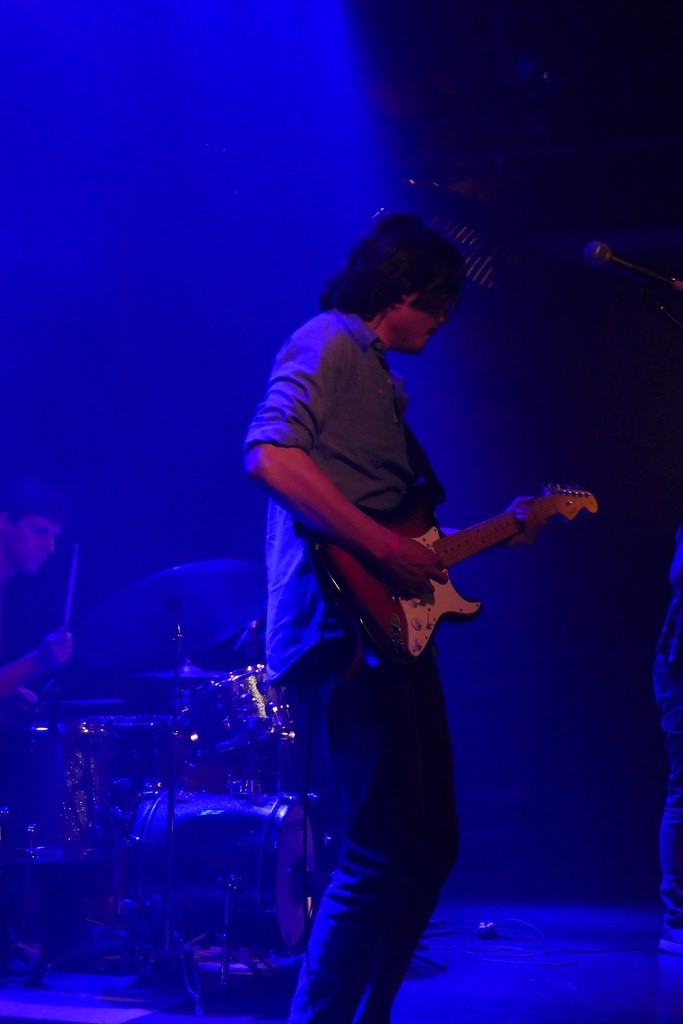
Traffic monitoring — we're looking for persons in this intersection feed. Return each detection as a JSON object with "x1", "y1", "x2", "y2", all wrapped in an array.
[
  {"x1": 651, "y1": 519, "x2": 683, "y2": 953},
  {"x1": 0, "y1": 500, "x2": 73, "y2": 838},
  {"x1": 243, "y1": 214, "x2": 471, "y2": 1023}
]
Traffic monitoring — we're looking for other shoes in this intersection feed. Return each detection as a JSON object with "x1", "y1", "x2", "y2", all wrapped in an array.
[{"x1": 659, "y1": 922, "x2": 683, "y2": 955}]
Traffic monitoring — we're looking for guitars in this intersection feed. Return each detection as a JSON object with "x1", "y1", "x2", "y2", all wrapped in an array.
[{"x1": 301, "y1": 482, "x2": 605, "y2": 682}]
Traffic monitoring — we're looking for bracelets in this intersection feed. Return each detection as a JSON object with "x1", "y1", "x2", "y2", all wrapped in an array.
[{"x1": 385, "y1": 549, "x2": 393, "y2": 564}]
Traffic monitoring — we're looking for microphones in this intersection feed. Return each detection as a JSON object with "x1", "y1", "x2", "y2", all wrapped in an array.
[{"x1": 583, "y1": 241, "x2": 683, "y2": 291}]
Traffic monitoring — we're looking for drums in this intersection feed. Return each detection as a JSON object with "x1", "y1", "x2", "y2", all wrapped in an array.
[
  {"x1": 0, "y1": 845, "x2": 138, "y2": 952},
  {"x1": 117, "y1": 780, "x2": 323, "y2": 956},
  {"x1": 170, "y1": 662, "x2": 306, "y2": 768}
]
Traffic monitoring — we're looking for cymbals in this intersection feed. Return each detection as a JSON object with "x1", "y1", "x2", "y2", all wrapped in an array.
[{"x1": 126, "y1": 665, "x2": 236, "y2": 687}]
